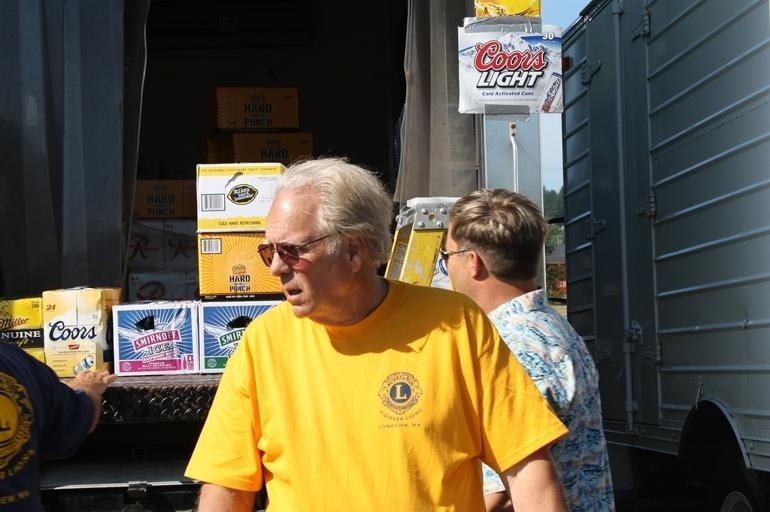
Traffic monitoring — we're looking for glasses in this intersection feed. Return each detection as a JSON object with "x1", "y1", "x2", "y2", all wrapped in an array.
[
  {"x1": 439, "y1": 247, "x2": 469, "y2": 260},
  {"x1": 257, "y1": 235, "x2": 330, "y2": 267}
]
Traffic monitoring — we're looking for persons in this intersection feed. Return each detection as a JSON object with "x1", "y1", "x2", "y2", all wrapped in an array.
[
  {"x1": 0, "y1": 341, "x2": 118, "y2": 512},
  {"x1": 443, "y1": 187, "x2": 616, "y2": 512},
  {"x1": 184, "y1": 154, "x2": 572, "y2": 512}
]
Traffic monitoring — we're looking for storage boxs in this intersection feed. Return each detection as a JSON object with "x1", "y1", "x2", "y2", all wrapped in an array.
[
  {"x1": 2, "y1": 296, "x2": 43, "y2": 377},
  {"x1": 187, "y1": 273, "x2": 206, "y2": 301},
  {"x1": 43, "y1": 284, "x2": 123, "y2": 378},
  {"x1": 110, "y1": 299, "x2": 199, "y2": 376},
  {"x1": 198, "y1": 301, "x2": 283, "y2": 375},
  {"x1": 195, "y1": 160, "x2": 289, "y2": 234},
  {"x1": 208, "y1": 86, "x2": 300, "y2": 134},
  {"x1": 202, "y1": 133, "x2": 312, "y2": 168},
  {"x1": 133, "y1": 179, "x2": 195, "y2": 219},
  {"x1": 161, "y1": 219, "x2": 195, "y2": 270},
  {"x1": 128, "y1": 271, "x2": 185, "y2": 303},
  {"x1": 196, "y1": 230, "x2": 285, "y2": 295},
  {"x1": 125, "y1": 220, "x2": 163, "y2": 270}
]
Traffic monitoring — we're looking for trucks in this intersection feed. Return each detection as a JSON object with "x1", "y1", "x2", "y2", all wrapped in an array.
[{"x1": 556, "y1": 2, "x2": 770, "y2": 511}]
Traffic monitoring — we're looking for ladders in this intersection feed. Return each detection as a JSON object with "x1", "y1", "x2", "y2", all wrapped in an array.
[{"x1": 382, "y1": 201, "x2": 459, "y2": 294}]
